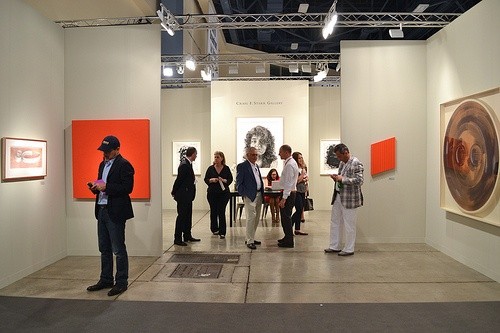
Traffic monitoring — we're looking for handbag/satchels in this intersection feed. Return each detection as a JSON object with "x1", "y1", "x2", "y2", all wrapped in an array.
[{"x1": 303, "y1": 196, "x2": 314, "y2": 211}]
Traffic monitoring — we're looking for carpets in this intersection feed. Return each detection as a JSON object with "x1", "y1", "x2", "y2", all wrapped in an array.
[{"x1": 0, "y1": 294, "x2": 500, "y2": 333}]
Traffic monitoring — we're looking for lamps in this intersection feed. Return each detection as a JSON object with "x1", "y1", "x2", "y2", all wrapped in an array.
[
  {"x1": 389, "y1": 23, "x2": 404, "y2": 38},
  {"x1": 229, "y1": 64, "x2": 240, "y2": 75},
  {"x1": 163, "y1": 64, "x2": 174, "y2": 77},
  {"x1": 200, "y1": 65, "x2": 214, "y2": 82},
  {"x1": 412, "y1": 4, "x2": 429, "y2": 13},
  {"x1": 185, "y1": 56, "x2": 197, "y2": 71},
  {"x1": 302, "y1": 62, "x2": 312, "y2": 72},
  {"x1": 297, "y1": 4, "x2": 309, "y2": 14},
  {"x1": 291, "y1": 43, "x2": 298, "y2": 51},
  {"x1": 156, "y1": 6, "x2": 178, "y2": 36},
  {"x1": 288, "y1": 62, "x2": 298, "y2": 73},
  {"x1": 321, "y1": 0, "x2": 338, "y2": 39},
  {"x1": 256, "y1": 63, "x2": 266, "y2": 74}
]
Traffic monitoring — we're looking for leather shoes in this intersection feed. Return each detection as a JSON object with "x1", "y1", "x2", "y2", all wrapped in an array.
[
  {"x1": 87, "y1": 279, "x2": 114, "y2": 291},
  {"x1": 108, "y1": 282, "x2": 125, "y2": 296}
]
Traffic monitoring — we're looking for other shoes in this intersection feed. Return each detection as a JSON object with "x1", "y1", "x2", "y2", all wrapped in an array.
[
  {"x1": 213, "y1": 230, "x2": 219, "y2": 235},
  {"x1": 277, "y1": 239, "x2": 294, "y2": 248},
  {"x1": 174, "y1": 241, "x2": 188, "y2": 246},
  {"x1": 245, "y1": 240, "x2": 261, "y2": 245},
  {"x1": 247, "y1": 243, "x2": 257, "y2": 249},
  {"x1": 220, "y1": 234, "x2": 225, "y2": 239},
  {"x1": 184, "y1": 237, "x2": 200, "y2": 242},
  {"x1": 338, "y1": 251, "x2": 354, "y2": 256},
  {"x1": 294, "y1": 230, "x2": 308, "y2": 235},
  {"x1": 301, "y1": 219, "x2": 306, "y2": 222},
  {"x1": 324, "y1": 248, "x2": 341, "y2": 253}
]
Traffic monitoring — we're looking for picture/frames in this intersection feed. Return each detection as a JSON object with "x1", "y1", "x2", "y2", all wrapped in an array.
[
  {"x1": 318, "y1": 139, "x2": 342, "y2": 176},
  {"x1": 172, "y1": 140, "x2": 202, "y2": 176},
  {"x1": 1, "y1": 137, "x2": 48, "y2": 181}
]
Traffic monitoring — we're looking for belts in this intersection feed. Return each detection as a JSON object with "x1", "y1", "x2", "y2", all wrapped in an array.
[{"x1": 336, "y1": 191, "x2": 341, "y2": 194}]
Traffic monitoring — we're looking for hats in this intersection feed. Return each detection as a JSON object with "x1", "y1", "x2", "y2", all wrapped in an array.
[{"x1": 97, "y1": 135, "x2": 121, "y2": 153}]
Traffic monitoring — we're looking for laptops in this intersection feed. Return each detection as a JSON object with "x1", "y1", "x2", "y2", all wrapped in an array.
[{"x1": 271, "y1": 181, "x2": 280, "y2": 190}]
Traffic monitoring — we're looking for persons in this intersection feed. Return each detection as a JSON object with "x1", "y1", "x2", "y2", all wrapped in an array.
[
  {"x1": 236, "y1": 146, "x2": 265, "y2": 250},
  {"x1": 171, "y1": 147, "x2": 201, "y2": 246},
  {"x1": 87, "y1": 136, "x2": 136, "y2": 296},
  {"x1": 278, "y1": 145, "x2": 298, "y2": 248},
  {"x1": 292, "y1": 152, "x2": 309, "y2": 235},
  {"x1": 204, "y1": 151, "x2": 233, "y2": 239},
  {"x1": 324, "y1": 143, "x2": 364, "y2": 256},
  {"x1": 262, "y1": 168, "x2": 282, "y2": 222}
]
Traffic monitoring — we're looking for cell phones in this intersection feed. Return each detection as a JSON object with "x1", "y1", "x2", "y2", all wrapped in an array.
[{"x1": 87, "y1": 183, "x2": 95, "y2": 188}]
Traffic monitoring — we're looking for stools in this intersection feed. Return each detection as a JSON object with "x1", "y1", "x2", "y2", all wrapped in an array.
[{"x1": 235, "y1": 203, "x2": 245, "y2": 222}]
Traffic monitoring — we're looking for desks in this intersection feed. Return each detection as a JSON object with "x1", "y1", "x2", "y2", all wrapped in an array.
[{"x1": 229, "y1": 190, "x2": 283, "y2": 227}]
